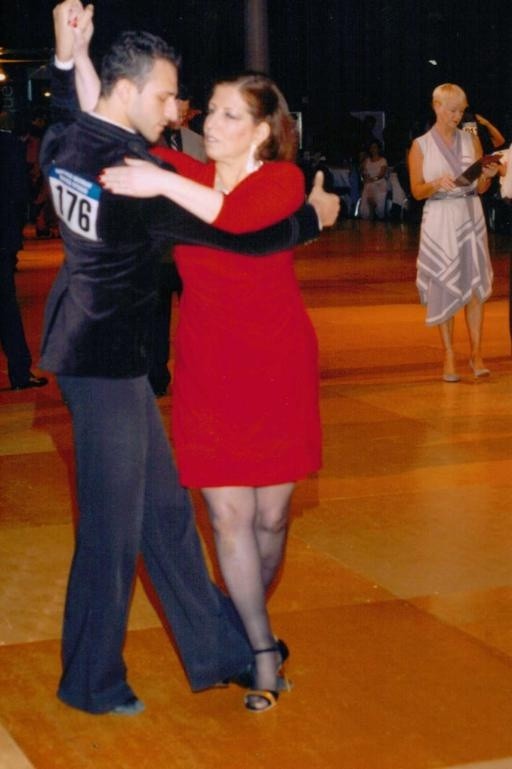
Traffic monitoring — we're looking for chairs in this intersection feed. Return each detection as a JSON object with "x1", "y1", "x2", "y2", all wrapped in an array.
[{"x1": 384, "y1": 173, "x2": 411, "y2": 235}]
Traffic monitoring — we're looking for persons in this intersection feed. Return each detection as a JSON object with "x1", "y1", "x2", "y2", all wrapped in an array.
[
  {"x1": 407, "y1": 84, "x2": 512, "y2": 382},
  {"x1": 152, "y1": 70, "x2": 210, "y2": 165},
  {"x1": 359, "y1": 142, "x2": 388, "y2": 219},
  {"x1": 1, "y1": 131, "x2": 48, "y2": 389}
]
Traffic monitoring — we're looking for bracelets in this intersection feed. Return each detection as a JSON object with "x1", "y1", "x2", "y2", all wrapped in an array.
[{"x1": 374, "y1": 176, "x2": 378, "y2": 181}]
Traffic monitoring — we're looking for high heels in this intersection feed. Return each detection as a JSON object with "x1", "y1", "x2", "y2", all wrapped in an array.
[
  {"x1": 243, "y1": 638, "x2": 289, "y2": 713},
  {"x1": 442, "y1": 359, "x2": 490, "y2": 382}
]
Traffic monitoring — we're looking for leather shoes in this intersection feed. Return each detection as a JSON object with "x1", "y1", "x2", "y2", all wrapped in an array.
[
  {"x1": 114, "y1": 695, "x2": 144, "y2": 714},
  {"x1": 10, "y1": 372, "x2": 47, "y2": 390},
  {"x1": 216, "y1": 657, "x2": 293, "y2": 692}
]
